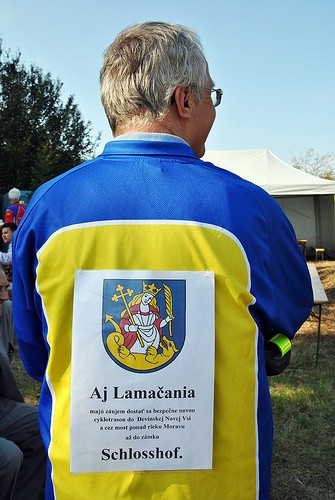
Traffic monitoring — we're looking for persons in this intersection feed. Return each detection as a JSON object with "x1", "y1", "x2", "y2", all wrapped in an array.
[
  {"x1": 0, "y1": 187, "x2": 27, "y2": 283},
  {"x1": 0, "y1": 268, "x2": 50, "y2": 500},
  {"x1": 10, "y1": 20, "x2": 314, "y2": 500}
]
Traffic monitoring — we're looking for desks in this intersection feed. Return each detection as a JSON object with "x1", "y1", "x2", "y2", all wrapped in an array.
[
  {"x1": 308, "y1": 300, "x2": 327, "y2": 366},
  {"x1": 297, "y1": 239, "x2": 309, "y2": 259}
]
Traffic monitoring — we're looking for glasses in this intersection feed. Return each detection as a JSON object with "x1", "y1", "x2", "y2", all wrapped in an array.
[{"x1": 170, "y1": 85, "x2": 223, "y2": 107}]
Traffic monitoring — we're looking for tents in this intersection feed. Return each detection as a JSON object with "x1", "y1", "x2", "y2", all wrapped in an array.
[{"x1": 197, "y1": 149, "x2": 335, "y2": 261}]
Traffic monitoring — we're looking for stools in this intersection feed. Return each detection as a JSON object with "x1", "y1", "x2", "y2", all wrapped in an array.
[{"x1": 310, "y1": 248, "x2": 325, "y2": 260}]
